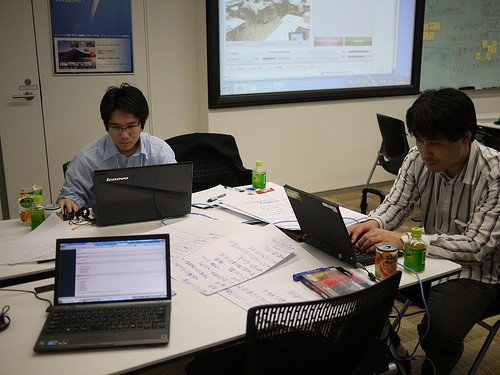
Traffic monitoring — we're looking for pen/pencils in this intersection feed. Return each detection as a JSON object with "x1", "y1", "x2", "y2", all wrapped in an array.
[{"x1": 207, "y1": 193, "x2": 226, "y2": 202}]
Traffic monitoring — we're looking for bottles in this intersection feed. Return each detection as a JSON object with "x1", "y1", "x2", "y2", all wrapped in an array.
[
  {"x1": 403, "y1": 227, "x2": 427, "y2": 274},
  {"x1": 252, "y1": 161, "x2": 266, "y2": 190}
]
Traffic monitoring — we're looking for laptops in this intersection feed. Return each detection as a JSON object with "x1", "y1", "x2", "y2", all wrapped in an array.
[
  {"x1": 91, "y1": 161, "x2": 193, "y2": 226},
  {"x1": 283, "y1": 184, "x2": 404, "y2": 267},
  {"x1": 33, "y1": 232, "x2": 172, "y2": 352}
]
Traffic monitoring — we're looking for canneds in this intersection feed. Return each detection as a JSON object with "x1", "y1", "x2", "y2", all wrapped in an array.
[{"x1": 376, "y1": 244, "x2": 398, "y2": 281}]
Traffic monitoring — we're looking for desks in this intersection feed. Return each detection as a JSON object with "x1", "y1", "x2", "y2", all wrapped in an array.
[{"x1": 0, "y1": 181, "x2": 462, "y2": 375}]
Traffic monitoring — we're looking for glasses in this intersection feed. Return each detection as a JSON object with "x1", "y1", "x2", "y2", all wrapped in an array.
[{"x1": 107, "y1": 118, "x2": 143, "y2": 134}]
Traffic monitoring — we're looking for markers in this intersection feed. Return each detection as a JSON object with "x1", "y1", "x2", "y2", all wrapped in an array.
[{"x1": 293, "y1": 265, "x2": 334, "y2": 281}]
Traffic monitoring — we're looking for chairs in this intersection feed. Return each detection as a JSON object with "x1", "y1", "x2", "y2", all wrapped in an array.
[
  {"x1": 163, "y1": 132, "x2": 253, "y2": 193},
  {"x1": 359, "y1": 113, "x2": 411, "y2": 215},
  {"x1": 391, "y1": 281, "x2": 500, "y2": 375},
  {"x1": 184, "y1": 271, "x2": 403, "y2": 375}
]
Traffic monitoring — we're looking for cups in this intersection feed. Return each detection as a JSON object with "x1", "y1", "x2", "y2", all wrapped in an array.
[{"x1": 30, "y1": 205, "x2": 45, "y2": 229}]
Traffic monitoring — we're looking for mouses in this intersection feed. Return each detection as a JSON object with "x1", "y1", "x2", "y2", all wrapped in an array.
[{"x1": 62, "y1": 205, "x2": 73, "y2": 220}]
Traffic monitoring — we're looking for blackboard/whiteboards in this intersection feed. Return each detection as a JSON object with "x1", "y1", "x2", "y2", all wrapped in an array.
[{"x1": 418, "y1": 0, "x2": 500, "y2": 96}]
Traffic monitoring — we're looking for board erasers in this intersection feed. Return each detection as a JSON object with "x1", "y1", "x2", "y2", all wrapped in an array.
[{"x1": 460, "y1": 86, "x2": 475, "y2": 90}]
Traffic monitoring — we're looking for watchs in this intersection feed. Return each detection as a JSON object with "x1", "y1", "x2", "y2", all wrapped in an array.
[{"x1": 401, "y1": 233, "x2": 409, "y2": 243}]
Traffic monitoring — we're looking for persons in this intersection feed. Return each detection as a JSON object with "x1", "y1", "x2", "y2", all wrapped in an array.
[
  {"x1": 344, "y1": 89, "x2": 500, "y2": 375},
  {"x1": 57, "y1": 82, "x2": 177, "y2": 215}
]
